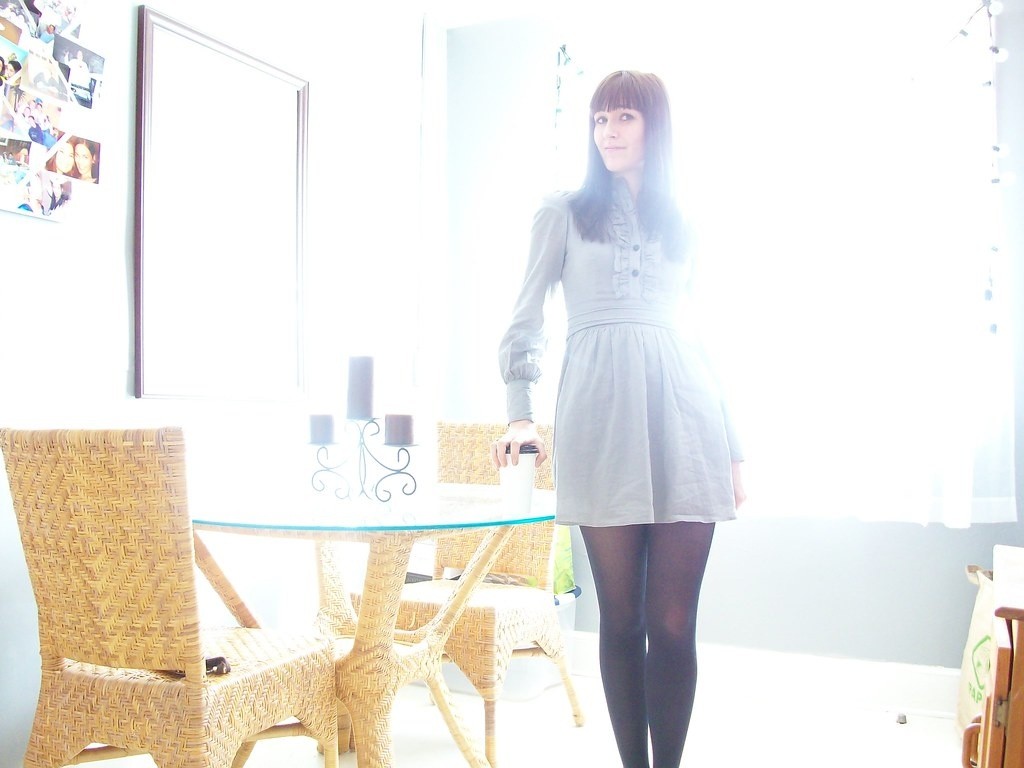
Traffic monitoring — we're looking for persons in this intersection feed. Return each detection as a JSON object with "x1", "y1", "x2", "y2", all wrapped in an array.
[
  {"x1": 2, "y1": 2, "x2": 98, "y2": 219},
  {"x1": 491, "y1": 69, "x2": 747, "y2": 767}
]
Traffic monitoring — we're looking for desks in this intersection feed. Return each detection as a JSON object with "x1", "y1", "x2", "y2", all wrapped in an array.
[{"x1": 194, "y1": 480, "x2": 555, "y2": 768}]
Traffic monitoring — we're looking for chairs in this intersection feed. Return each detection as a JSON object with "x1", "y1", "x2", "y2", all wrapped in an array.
[
  {"x1": 336, "y1": 423, "x2": 585, "y2": 768},
  {"x1": 2, "y1": 425, "x2": 341, "y2": 768}
]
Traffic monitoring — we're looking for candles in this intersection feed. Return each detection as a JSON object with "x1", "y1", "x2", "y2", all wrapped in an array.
[
  {"x1": 345, "y1": 356, "x2": 373, "y2": 420},
  {"x1": 309, "y1": 414, "x2": 336, "y2": 445},
  {"x1": 385, "y1": 415, "x2": 412, "y2": 445}
]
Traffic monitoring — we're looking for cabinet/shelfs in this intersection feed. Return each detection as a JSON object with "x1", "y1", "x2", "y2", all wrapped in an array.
[{"x1": 959, "y1": 542, "x2": 1024, "y2": 768}]
